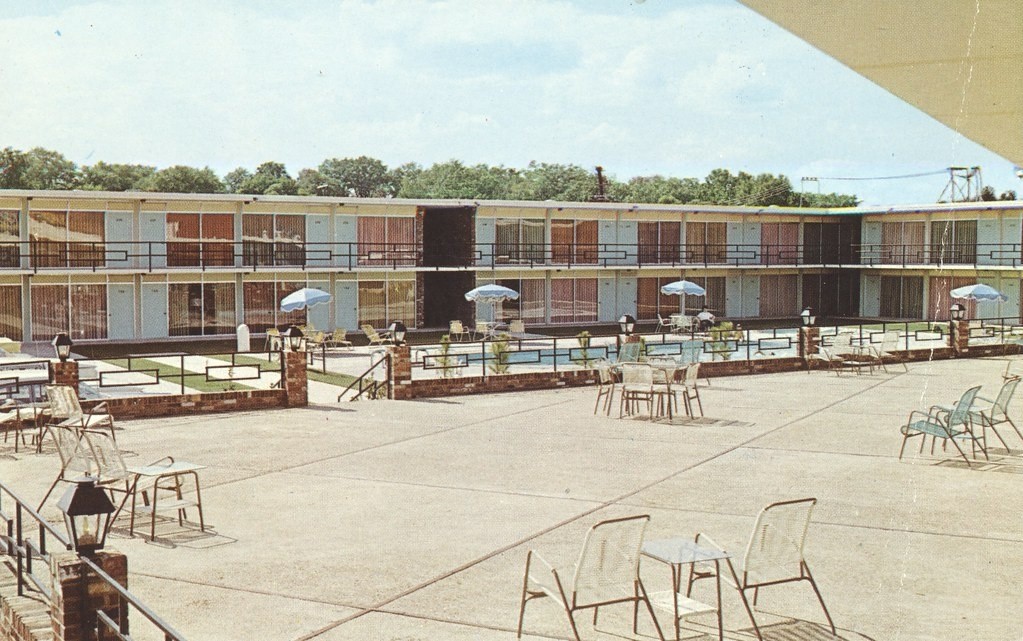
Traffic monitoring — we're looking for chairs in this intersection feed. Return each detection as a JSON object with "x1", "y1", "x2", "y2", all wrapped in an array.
[
  {"x1": 517, "y1": 514, "x2": 665, "y2": 641},
  {"x1": 0, "y1": 382, "x2": 209, "y2": 542},
  {"x1": 799, "y1": 327, "x2": 910, "y2": 377},
  {"x1": 592, "y1": 311, "x2": 712, "y2": 426},
  {"x1": 684, "y1": 496, "x2": 838, "y2": 641},
  {"x1": 263, "y1": 323, "x2": 355, "y2": 351},
  {"x1": 899, "y1": 385, "x2": 991, "y2": 468},
  {"x1": 447, "y1": 318, "x2": 527, "y2": 344},
  {"x1": 361, "y1": 323, "x2": 391, "y2": 347},
  {"x1": 944, "y1": 376, "x2": 1023, "y2": 454}
]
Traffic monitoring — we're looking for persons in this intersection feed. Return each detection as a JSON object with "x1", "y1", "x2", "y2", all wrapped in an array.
[
  {"x1": 693, "y1": 305, "x2": 715, "y2": 332},
  {"x1": 281, "y1": 324, "x2": 304, "y2": 347}
]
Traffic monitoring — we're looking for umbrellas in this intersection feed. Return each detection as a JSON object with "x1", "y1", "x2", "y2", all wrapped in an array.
[
  {"x1": 280, "y1": 285, "x2": 332, "y2": 349},
  {"x1": 464, "y1": 280, "x2": 520, "y2": 341},
  {"x1": 661, "y1": 278, "x2": 706, "y2": 332},
  {"x1": 949, "y1": 283, "x2": 1007, "y2": 324}
]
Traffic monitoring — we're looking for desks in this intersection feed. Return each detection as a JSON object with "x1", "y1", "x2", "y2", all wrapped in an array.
[
  {"x1": 605, "y1": 361, "x2": 690, "y2": 421},
  {"x1": 942, "y1": 404, "x2": 991, "y2": 462},
  {"x1": 632, "y1": 537, "x2": 732, "y2": 641},
  {"x1": 834, "y1": 340, "x2": 884, "y2": 374},
  {"x1": 475, "y1": 321, "x2": 496, "y2": 341}
]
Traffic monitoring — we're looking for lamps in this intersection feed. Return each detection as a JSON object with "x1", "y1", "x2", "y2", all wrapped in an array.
[
  {"x1": 387, "y1": 319, "x2": 408, "y2": 346},
  {"x1": 51, "y1": 330, "x2": 74, "y2": 363},
  {"x1": 282, "y1": 324, "x2": 304, "y2": 352},
  {"x1": 59, "y1": 471, "x2": 116, "y2": 550},
  {"x1": 798, "y1": 305, "x2": 818, "y2": 328},
  {"x1": 948, "y1": 302, "x2": 967, "y2": 320},
  {"x1": 616, "y1": 313, "x2": 637, "y2": 336}
]
